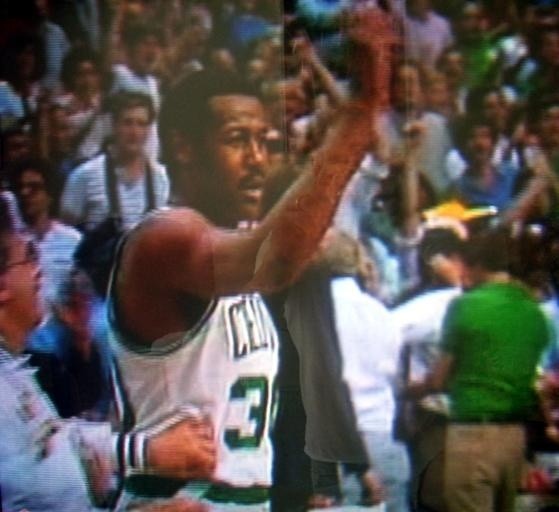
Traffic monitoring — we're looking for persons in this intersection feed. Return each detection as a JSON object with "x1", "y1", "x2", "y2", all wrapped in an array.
[{"x1": 0, "y1": 0, "x2": 559, "y2": 512}]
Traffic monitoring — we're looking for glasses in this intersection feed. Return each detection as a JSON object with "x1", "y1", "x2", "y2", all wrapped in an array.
[{"x1": 5, "y1": 241, "x2": 38, "y2": 269}]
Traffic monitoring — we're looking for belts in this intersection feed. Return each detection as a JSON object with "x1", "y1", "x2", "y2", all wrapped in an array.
[{"x1": 470, "y1": 413, "x2": 518, "y2": 424}]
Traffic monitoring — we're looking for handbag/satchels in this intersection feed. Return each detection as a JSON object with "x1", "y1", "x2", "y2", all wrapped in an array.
[{"x1": 73, "y1": 216, "x2": 126, "y2": 298}]
